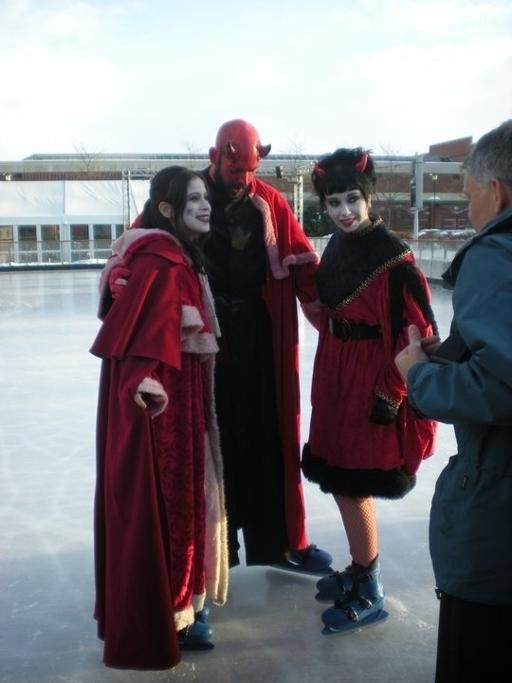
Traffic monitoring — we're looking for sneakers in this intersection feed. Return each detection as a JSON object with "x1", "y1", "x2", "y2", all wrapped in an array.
[
  {"x1": 176, "y1": 617, "x2": 216, "y2": 644},
  {"x1": 276, "y1": 544, "x2": 333, "y2": 569},
  {"x1": 194, "y1": 603, "x2": 211, "y2": 622}
]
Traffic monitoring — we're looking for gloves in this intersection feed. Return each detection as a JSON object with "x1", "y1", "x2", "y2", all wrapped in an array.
[{"x1": 369, "y1": 396, "x2": 398, "y2": 425}]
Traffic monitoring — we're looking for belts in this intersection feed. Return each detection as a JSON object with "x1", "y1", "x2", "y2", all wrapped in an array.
[{"x1": 327, "y1": 316, "x2": 382, "y2": 342}]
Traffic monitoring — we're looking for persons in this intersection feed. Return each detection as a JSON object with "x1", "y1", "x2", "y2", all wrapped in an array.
[
  {"x1": 90, "y1": 166, "x2": 229, "y2": 668},
  {"x1": 98, "y1": 120, "x2": 331, "y2": 570},
  {"x1": 302, "y1": 148, "x2": 441, "y2": 628},
  {"x1": 394, "y1": 119, "x2": 512, "y2": 683}
]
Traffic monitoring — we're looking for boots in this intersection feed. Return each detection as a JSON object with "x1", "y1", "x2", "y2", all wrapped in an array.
[
  {"x1": 322, "y1": 553, "x2": 386, "y2": 628},
  {"x1": 316, "y1": 564, "x2": 357, "y2": 594}
]
